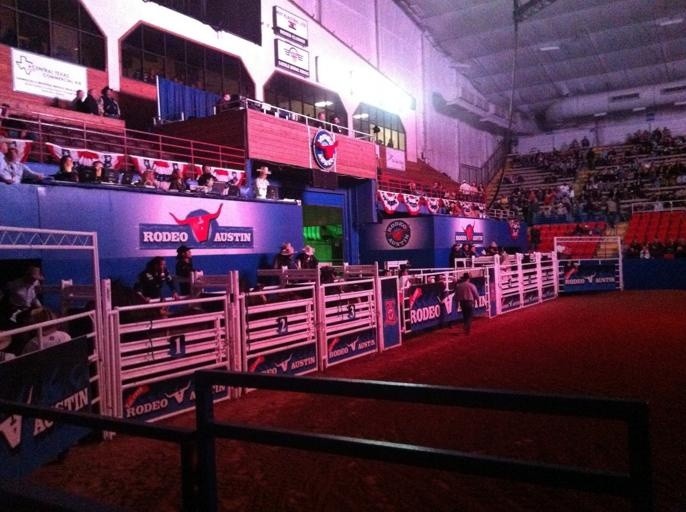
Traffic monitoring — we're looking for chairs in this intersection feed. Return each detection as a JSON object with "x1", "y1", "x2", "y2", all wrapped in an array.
[
  {"x1": 530, "y1": 208, "x2": 686, "y2": 260},
  {"x1": 0, "y1": 111, "x2": 173, "y2": 161}
]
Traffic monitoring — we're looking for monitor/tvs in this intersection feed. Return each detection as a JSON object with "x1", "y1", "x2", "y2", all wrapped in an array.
[
  {"x1": 120, "y1": 173, "x2": 134, "y2": 184},
  {"x1": 78, "y1": 166, "x2": 96, "y2": 182},
  {"x1": 212, "y1": 180, "x2": 226, "y2": 196},
  {"x1": 101, "y1": 168, "x2": 119, "y2": 183}
]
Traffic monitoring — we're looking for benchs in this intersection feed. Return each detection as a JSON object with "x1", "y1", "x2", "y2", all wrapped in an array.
[
  {"x1": 488, "y1": 138, "x2": 686, "y2": 208},
  {"x1": 378, "y1": 150, "x2": 476, "y2": 199}
]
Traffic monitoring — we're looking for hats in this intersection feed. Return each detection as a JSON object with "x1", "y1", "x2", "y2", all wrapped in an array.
[
  {"x1": 301, "y1": 244, "x2": 314, "y2": 256},
  {"x1": 175, "y1": 245, "x2": 195, "y2": 258},
  {"x1": 31, "y1": 307, "x2": 58, "y2": 335},
  {"x1": 281, "y1": 246, "x2": 297, "y2": 256},
  {"x1": 101, "y1": 86, "x2": 113, "y2": 95},
  {"x1": 257, "y1": 166, "x2": 272, "y2": 175},
  {"x1": 25, "y1": 266, "x2": 46, "y2": 280}
]
